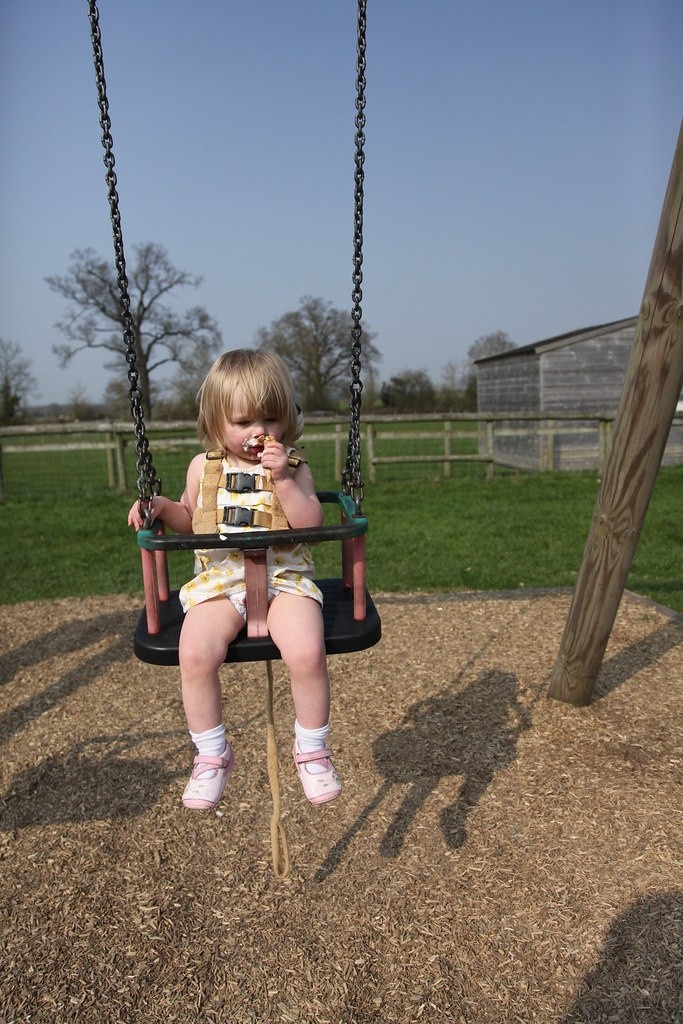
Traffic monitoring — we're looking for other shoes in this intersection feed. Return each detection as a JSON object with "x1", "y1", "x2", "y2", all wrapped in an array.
[
  {"x1": 293, "y1": 740, "x2": 342, "y2": 804},
  {"x1": 182, "y1": 741, "x2": 234, "y2": 809}
]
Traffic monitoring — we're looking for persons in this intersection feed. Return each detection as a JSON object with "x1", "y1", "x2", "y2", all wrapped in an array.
[{"x1": 128, "y1": 347, "x2": 344, "y2": 809}]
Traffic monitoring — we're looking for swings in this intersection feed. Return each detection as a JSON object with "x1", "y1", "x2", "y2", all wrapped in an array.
[{"x1": 89, "y1": 1, "x2": 381, "y2": 664}]
